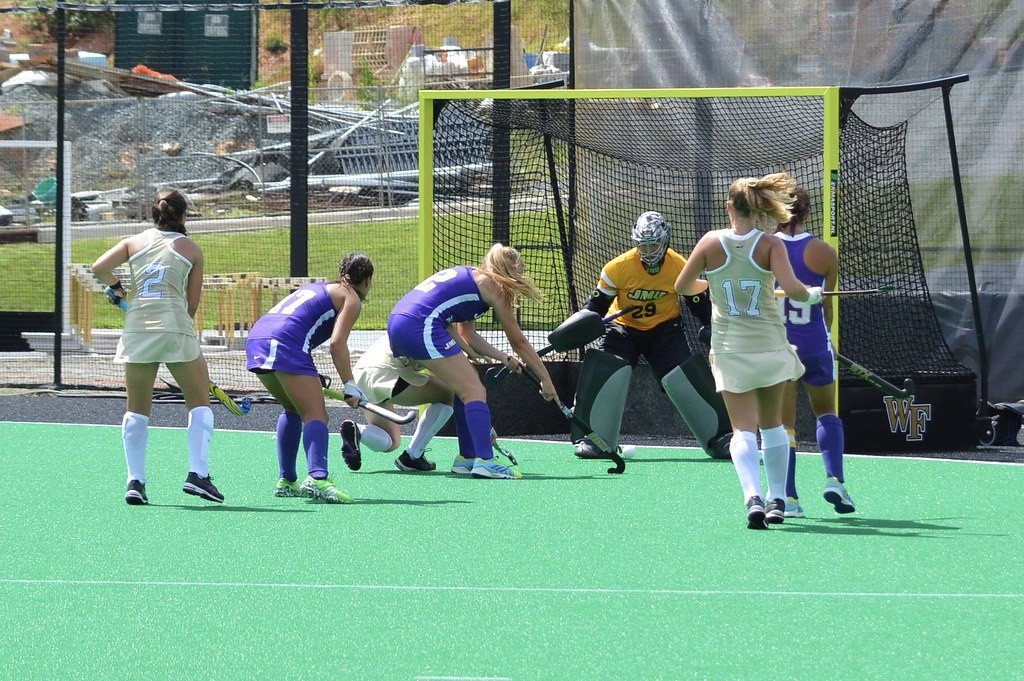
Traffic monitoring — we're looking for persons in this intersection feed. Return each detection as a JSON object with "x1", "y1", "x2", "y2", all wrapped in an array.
[
  {"x1": 570, "y1": 211, "x2": 733, "y2": 459},
  {"x1": 247, "y1": 252, "x2": 374, "y2": 502},
  {"x1": 674, "y1": 172, "x2": 824, "y2": 529},
  {"x1": 91, "y1": 188, "x2": 225, "y2": 504},
  {"x1": 387, "y1": 244, "x2": 561, "y2": 479},
  {"x1": 340, "y1": 334, "x2": 454, "y2": 471},
  {"x1": 772, "y1": 189, "x2": 855, "y2": 517}
]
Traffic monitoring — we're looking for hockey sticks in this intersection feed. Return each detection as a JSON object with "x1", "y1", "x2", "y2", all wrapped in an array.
[
  {"x1": 506, "y1": 356, "x2": 626, "y2": 473},
  {"x1": 773, "y1": 286, "x2": 897, "y2": 295},
  {"x1": 105, "y1": 285, "x2": 252, "y2": 417},
  {"x1": 833, "y1": 352, "x2": 915, "y2": 401},
  {"x1": 493, "y1": 440, "x2": 518, "y2": 466},
  {"x1": 322, "y1": 386, "x2": 417, "y2": 425},
  {"x1": 484, "y1": 304, "x2": 637, "y2": 383}
]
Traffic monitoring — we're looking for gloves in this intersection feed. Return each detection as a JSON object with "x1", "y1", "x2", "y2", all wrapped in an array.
[{"x1": 803, "y1": 286, "x2": 827, "y2": 305}]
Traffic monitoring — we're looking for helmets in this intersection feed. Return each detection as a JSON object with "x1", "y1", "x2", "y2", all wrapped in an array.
[{"x1": 631, "y1": 211, "x2": 671, "y2": 267}]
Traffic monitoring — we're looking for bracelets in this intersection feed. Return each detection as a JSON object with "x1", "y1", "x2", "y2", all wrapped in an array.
[{"x1": 109, "y1": 280, "x2": 121, "y2": 289}]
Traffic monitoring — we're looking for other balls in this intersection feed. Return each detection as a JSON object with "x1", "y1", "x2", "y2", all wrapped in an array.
[{"x1": 623, "y1": 445, "x2": 635, "y2": 458}]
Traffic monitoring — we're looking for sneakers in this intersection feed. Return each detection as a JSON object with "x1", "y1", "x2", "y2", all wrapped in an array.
[
  {"x1": 746, "y1": 496, "x2": 769, "y2": 530},
  {"x1": 300, "y1": 472, "x2": 353, "y2": 503},
  {"x1": 273, "y1": 477, "x2": 302, "y2": 497},
  {"x1": 575, "y1": 440, "x2": 600, "y2": 458},
  {"x1": 125, "y1": 479, "x2": 149, "y2": 506},
  {"x1": 822, "y1": 478, "x2": 856, "y2": 514},
  {"x1": 716, "y1": 433, "x2": 733, "y2": 457},
  {"x1": 763, "y1": 498, "x2": 785, "y2": 524},
  {"x1": 783, "y1": 497, "x2": 804, "y2": 517},
  {"x1": 338, "y1": 420, "x2": 362, "y2": 471},
  {"x1": 183, "y1": 472, "x2": 224, "y2": 503},
  {"x1": 451, "y1": 454, "x2": 475, "y2": 473},
  {"x1": 395, "y1": 447, "x2": 436, "y2": 471},
  {"x1": 469, "y1": 457, "x2": 517, "y2": 479}
]
